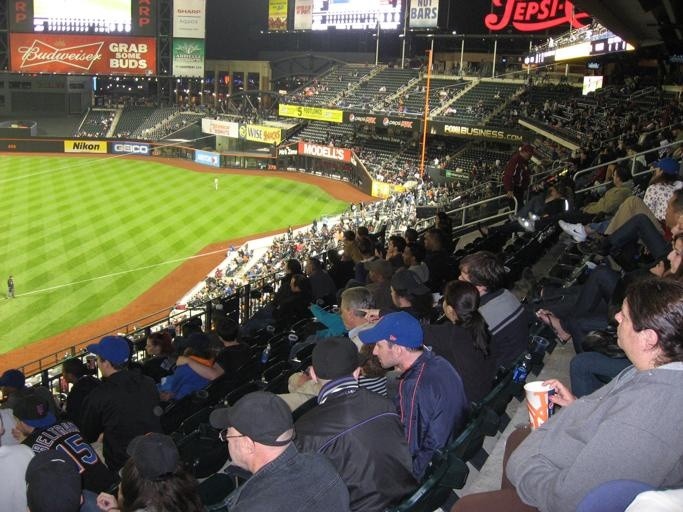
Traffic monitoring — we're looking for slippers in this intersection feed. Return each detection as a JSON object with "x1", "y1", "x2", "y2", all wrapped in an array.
[{"x1": 536, "y1": 309, "x2": 570, "y2": 344}]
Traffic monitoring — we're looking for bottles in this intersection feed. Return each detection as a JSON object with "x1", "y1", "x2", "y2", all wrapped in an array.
[
  {"x1": 260, "y1": 343, "x2": 270, "y2": 364},
  {"x1": 510, "y1": 353, "x2": 532, "y2": 384}
]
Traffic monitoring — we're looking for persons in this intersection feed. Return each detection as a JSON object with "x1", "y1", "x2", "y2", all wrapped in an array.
[{"x1": 0, "y1": 67, "x2": 683, "y2": 511}]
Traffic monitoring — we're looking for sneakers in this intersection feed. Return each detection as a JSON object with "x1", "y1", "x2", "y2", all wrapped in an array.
[
  {"x1": 559, "y1": 219, "x2": 587, "y2": 242},
  {"x1": 577, "y1": 237, "x2": 609, "y2": 256},
  {"x1": 522, "y1": 267, "x2": 542, "y2": 302}
]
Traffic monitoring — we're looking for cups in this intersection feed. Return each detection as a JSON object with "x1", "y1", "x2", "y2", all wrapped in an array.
[
  {"x1": 522, "y1": 381, "x2": 554, "y2": 432},
  {"x1": 531, "y1": 333, "x2": 549, "y2": 358}
]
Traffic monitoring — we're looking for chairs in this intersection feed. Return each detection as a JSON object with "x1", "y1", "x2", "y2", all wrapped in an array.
[
  {"x1": 79, "y1": 54, "x2": 682, "y2": 209},
  {"x1": 0, "y1": 205, "x2": 681, "y2": 512}
]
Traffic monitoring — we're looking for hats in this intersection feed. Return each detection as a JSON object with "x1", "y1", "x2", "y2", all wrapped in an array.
[
  {"x1": 210, "y1": 392, "x2": 296, "y2": 447},
  {"x1": 87, "y1": 336, "x2": 130, "y2": 365},
  {"x1": 654, "y1": 157, "x2": 680, "y2": 175},
  {"x1": 14, "y1": 395, "x2": 57, "y2": 428},
  {"x1": 26, "y1": 449, "x2": 82, "y2": 512},
  {"x1": 312, "y1": 337, "x2": 359, "y2": 379},
  {"x1": 358, "y1": 311, "x2": 424, "y2": 348}
]
